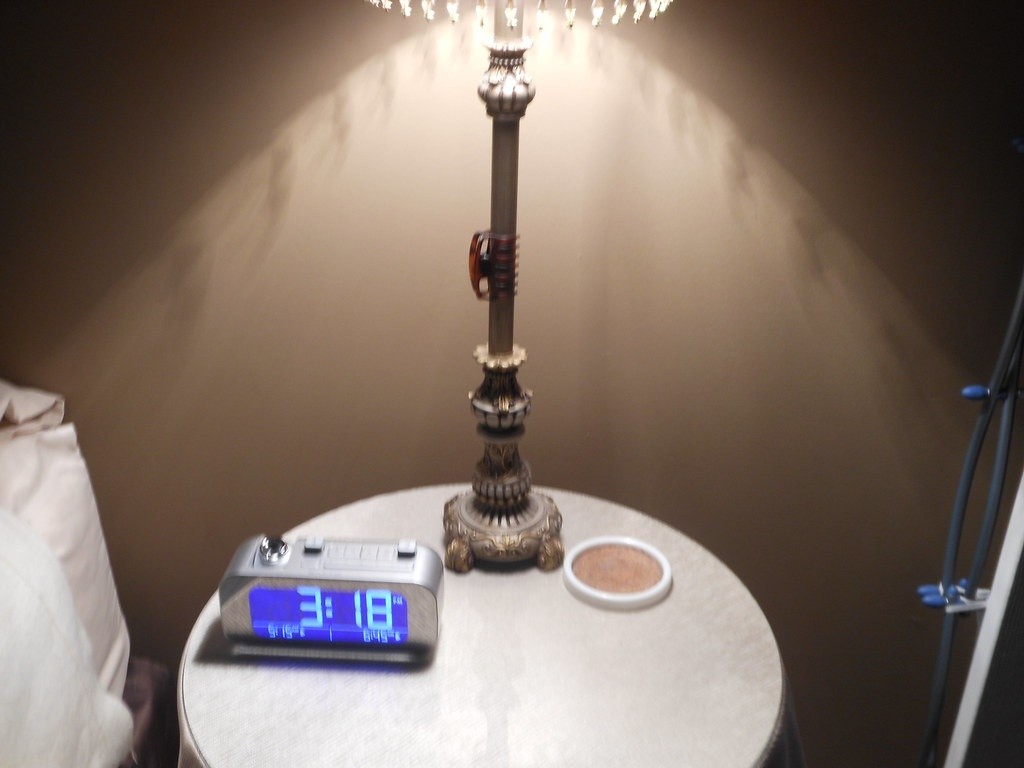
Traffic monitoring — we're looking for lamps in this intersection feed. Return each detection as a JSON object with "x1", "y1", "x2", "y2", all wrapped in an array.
[{"x1": 367, "y1": 0, "x2": 673, "y2": 575}]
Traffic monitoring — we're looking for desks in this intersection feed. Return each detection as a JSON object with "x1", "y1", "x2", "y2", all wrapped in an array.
[{"x1": 176, "y1": 482, "x2": 807, "y2": 768}]
